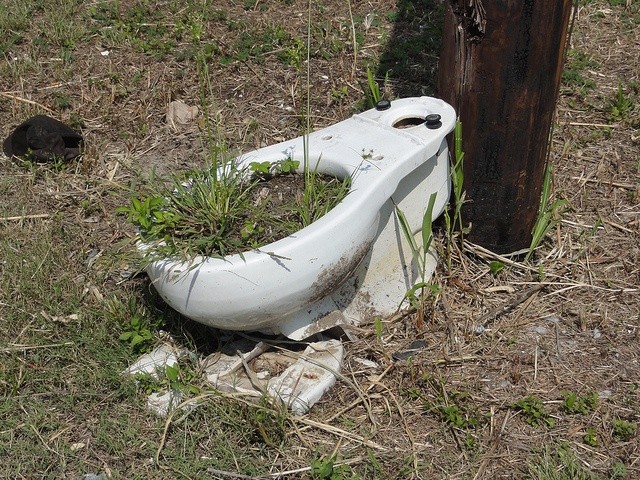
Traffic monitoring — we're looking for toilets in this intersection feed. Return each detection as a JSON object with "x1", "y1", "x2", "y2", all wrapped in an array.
[{"x1": 134, "y1": 95, "x2": 459, "y2": 343}]
